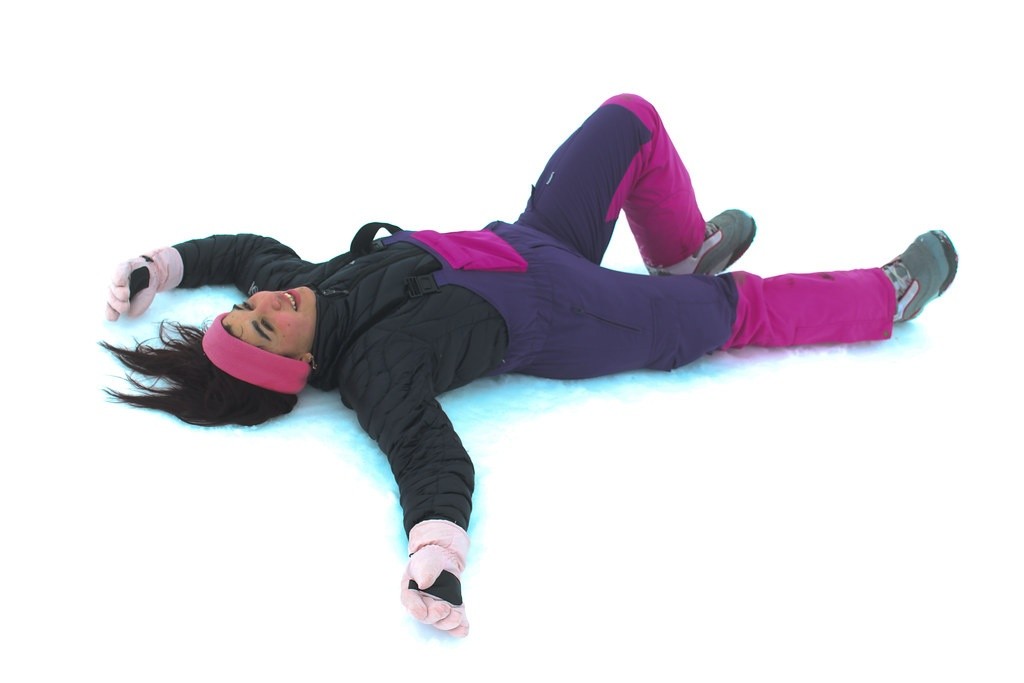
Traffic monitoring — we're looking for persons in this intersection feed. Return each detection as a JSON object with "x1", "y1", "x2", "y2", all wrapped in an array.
[{"x1": 100, "y1": 94, "x2": 959, "y2": 637}]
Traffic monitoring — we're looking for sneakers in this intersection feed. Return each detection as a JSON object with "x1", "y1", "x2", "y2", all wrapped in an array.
[
  {"x1": 646, "y1": 209, "x2": 756, "y2": 276},
  {"x1": 881, "y1": 229, "x2": 958, "y2": 321}
]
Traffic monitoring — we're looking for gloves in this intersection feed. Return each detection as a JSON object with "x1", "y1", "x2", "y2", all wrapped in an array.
[
  {"x1": 105, "y1": 245, "x2": 183, "y2": 321},
  {"x1": 401, "y1": 520, "x2": 468, "y2": 639}
]
